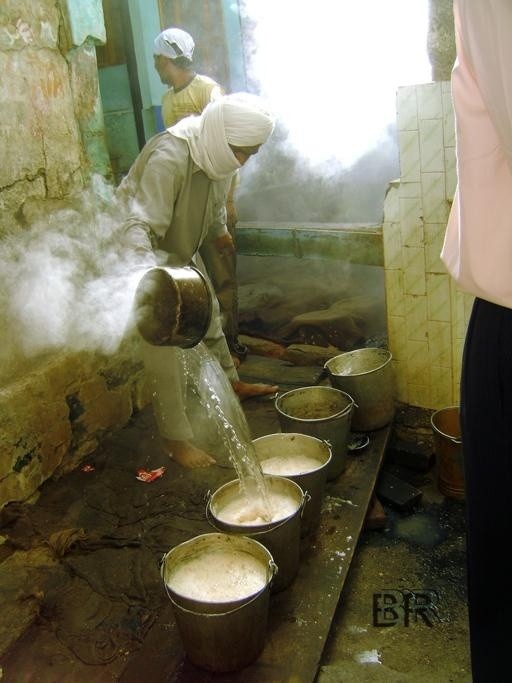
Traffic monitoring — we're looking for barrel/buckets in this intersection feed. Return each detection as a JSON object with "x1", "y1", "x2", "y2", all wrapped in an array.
[
  {"x1": 202, "y1": 477, "x2": 312, "y2": 595},
  {"x1": 325, "y1": 344, "x2": 395, "y2": 432},
  {"x1": 246, "y1": 432, "x2": 334, "y2": 536},
  {"x1": 157, "y1": 532, "x2": 278, "y2": 676},
  {"x1": 430, "y1": 405, "x2": 466, "y2": 497},
  {"x1": 270, "y1": 382, "x2": 360, "y2": 479}
]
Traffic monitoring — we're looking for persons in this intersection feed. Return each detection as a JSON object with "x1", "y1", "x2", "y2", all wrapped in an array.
[{"x1": 440, "y1": 0, "x2": 512, "y2": 683}]
